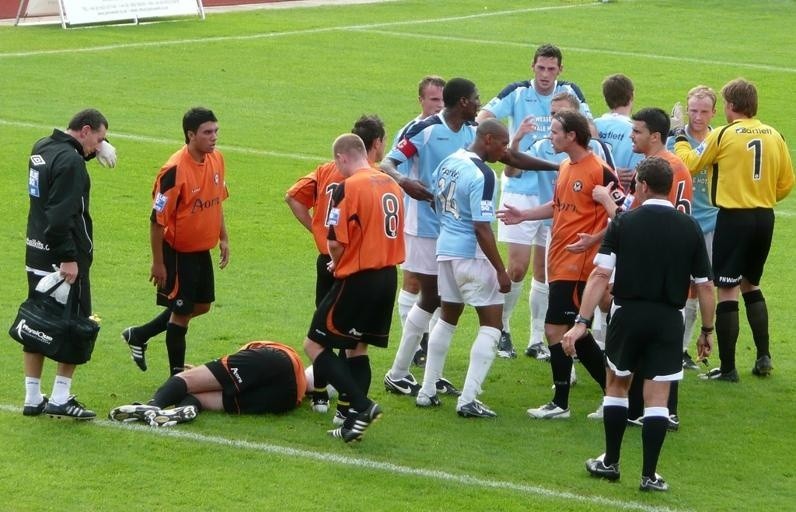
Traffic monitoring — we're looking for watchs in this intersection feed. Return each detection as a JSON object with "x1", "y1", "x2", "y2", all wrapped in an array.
[{"x1": 575, "y1": 315, "x2": 593, "y2": 330}]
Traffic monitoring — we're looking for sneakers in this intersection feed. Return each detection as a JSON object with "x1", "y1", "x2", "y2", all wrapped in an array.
[
  {"x1": 525, "y1": 342, "x2": 552, "y2": 360},
  {"x1": 436, "y1": 378, "x2": 462, "y2": 397},
  {"x1": 332, "y1": 409, "x2": 359, "y2": 426},
  {"x1": 122, "y1": 328, "x2": 149, "y2": 371},
  {"x1": 627, "y1": 413, "x2": 679, "y2": 431},
  {"x1": 45, "y1": 395, "x2": 98, "y2": 420},
  {"x1": 497, "y1": 332, "x2": 519, "y2": 358},
  {"x1": 587, "y1": 406, "x2": 605, "y2": 419},
  {"x1": 752, "y1": 355, "x2": 772, "y2": 377},
  {"x1": 527, "y1": 401, "x2": 570, "y2": 418},
  {"x1": 696, "y1": 367, "x2": 738, "y2": 382},
  {"x1": 309, "y1": 397, "x2": 330, "y2": 414},
  {"x1": 342, "y1": 403, "x2": 384, "y2": 442},
  {"x1": 552, "y1": 369, "x2": 578, "y2": 389},
  {"x1": 638, "y1": 473, "x2": 668, "y2": 493},
  {"x1": 414, "y1": 349, "x2": 428, "y2": 367},
  {"x1": 21, "y1": 397, "x2": 49, "y2": 417},
  {"x1": 108, "y1": 405, "x2": 160, "y2": 421},
  {"x1": 417, "y1": 391, "x2": 441, "y2": 407},
  {"x1": 326, "y1": 427, "x2": 363, "y2": 443},
  {"x1": 144, "y1": 405, "x2": 196, "y2": 428},
  {"x1": 681, "y1": 351, "x2": 701, "y2": 369},
  {"x1": 456, "y1": 395, "x2": 497, "y2": 418},
  {"x1": 383, "y1": 368, "x2": 422, "y2": 396},
  {"x1": 586, "y1": 457, "x2": 622, "y2": 482}
]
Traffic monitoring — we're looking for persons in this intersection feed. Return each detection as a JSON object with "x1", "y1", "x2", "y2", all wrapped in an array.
[
  {"x1": 108, "y1": 341, "x2": 308, "y2": 428},
  {"x1": 393, "y1": 72, "x2": 447, "y2": 368},
  {"x1": 303, "y1": 133, "x2": 406, "y2": 444},
  {"x1": 122, "y1": 106, "x2": 230, "y2": 377},
  {"x1": 475, "y1": 44, "x2": 632, "y2": 389},
  {"x1": 591, "y1": 107, "x2": 694, "y2": 431},
  {"x1": 671, "y1": 77, "x2": 796, "y2": 383},
  {"x1": 666, "y1": 85, "x2": 720, "y2": 371},
  {"x1": 592, "y1": 72, "x2": 647, "y2": 367},
  {"x1": 560, "y1": 154, "x2": 718, "y2": 492},
  {"x1": 379, "y1": 77, "x2": 563, "y2": 397},
  {"x1": 22, "y1": 108, "x2": 118, "y2": 421},
  {"x1": 504, "y1": 91, "x2": 617, "y2": 363},
  {"x1": 283, "y1": 112, "x2": 387, "y2": 425},
  {"x1": 493, "y1": 109, "x2": 627, "y2": 420},
  {"x1": 416, "y1": 117, "x2": 512, "y2": 420}
]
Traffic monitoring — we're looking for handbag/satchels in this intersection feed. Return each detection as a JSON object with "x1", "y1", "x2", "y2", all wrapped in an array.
[{"x1": 7, "y1": 281, "x2": 99, "y2": 365}]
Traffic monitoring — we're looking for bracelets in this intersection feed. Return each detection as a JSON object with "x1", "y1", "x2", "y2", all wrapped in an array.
[{"x1": 700, "y1": 326, "x2": 715, "y2": 335}]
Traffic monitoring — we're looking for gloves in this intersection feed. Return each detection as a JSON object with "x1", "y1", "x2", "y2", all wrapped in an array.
[
  {"x1": 93, "y1": 139, "x2": 120, "y2": 170},
  {"x1": 668, "y1": 103, "x2": 690, "y2": 141}
]
